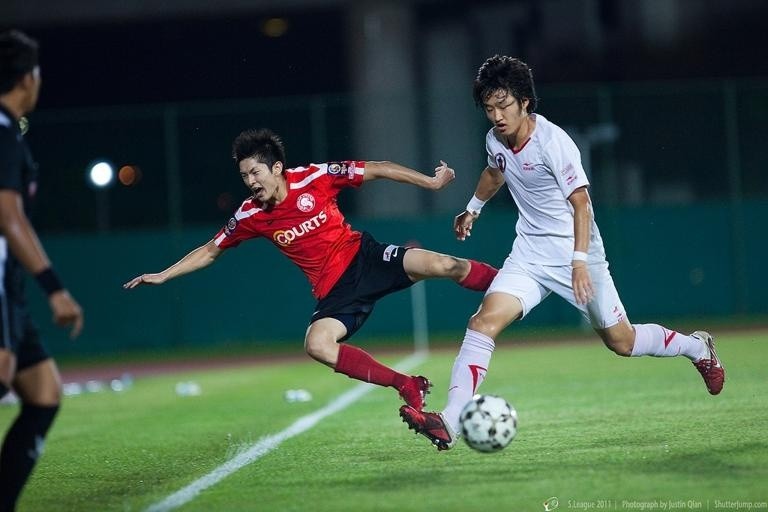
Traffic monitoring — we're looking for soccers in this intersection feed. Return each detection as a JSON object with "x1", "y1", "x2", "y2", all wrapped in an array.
[{"x1": 458, "y1": 394, "x2": 519, "y2": 453}]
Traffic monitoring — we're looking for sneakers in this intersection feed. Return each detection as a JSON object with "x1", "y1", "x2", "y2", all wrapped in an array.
[
  {"x1": 400, "y1": 405, "x2": 457, "y2": 451},
  {"x1": 398, "y1": 377, "x2": 432, "y2": 410},
  {"x1": 688, "y1": 329, "x2": 723, "y2": 396}
]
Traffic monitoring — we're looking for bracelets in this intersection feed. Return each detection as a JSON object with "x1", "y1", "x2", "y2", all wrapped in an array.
[
  {"x1": 572, "y1": 250, "x2": 588, "y2": 261},
  {"x1": 465, "y1": 195, "x2": 486, "y2": 217},
  {"x1": 33, "y1": 265, "x2": 64, "y2": 297}
]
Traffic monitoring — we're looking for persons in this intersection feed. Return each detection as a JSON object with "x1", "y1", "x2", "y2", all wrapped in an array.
[
  {"x1": 399, "y1": 54, "x2": 725, "y2": 450},
  {"x1": 1, "y1": 31, "x2": 84, "y2": 511},
  {"x1": 123, "y1": 130, "x2": 499, "y2": 412}
]
